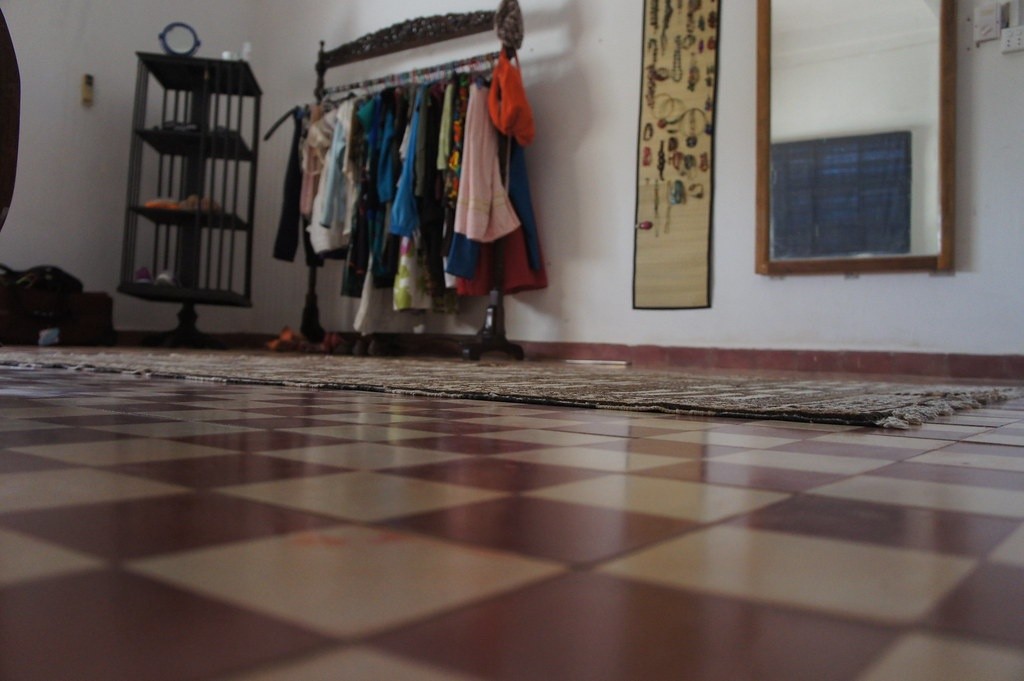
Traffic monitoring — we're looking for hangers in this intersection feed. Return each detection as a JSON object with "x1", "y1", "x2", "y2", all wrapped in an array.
[{"x1": 311, "y1": 49, "x2": 498, "y2": 105}]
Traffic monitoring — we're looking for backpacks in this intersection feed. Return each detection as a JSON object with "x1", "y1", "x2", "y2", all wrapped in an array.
[{"x1": 488, "y1": 51, "x2": 535, "y2": 146}]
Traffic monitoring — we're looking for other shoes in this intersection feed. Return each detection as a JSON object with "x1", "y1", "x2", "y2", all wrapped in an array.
[
  {"x1": 155, "y1": 271, "x2": 178, "y2": 286},
  {"x1": 135, "y1": 267, "x2": 151, "y2": 284},
  {"x1": 354, "y1": 337, "x2": 404, "y2": 356},
  {"x1": 324, "y1": 331, "x2": 355, "y2": 353}
]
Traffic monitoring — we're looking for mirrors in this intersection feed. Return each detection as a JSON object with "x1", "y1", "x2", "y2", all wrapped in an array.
[{"x1": 755, "y1": 0, "x2": 953, "y2": 277}]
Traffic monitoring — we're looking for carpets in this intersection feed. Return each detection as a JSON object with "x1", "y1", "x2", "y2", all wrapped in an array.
[{"x1": 0, "y1": 347, "x2": 1019, "y2": 430}]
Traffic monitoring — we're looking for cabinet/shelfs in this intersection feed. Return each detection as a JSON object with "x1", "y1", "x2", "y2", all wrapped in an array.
[{"x1": 114, "y1": 50, "x2": 262, "y2": 352}]
